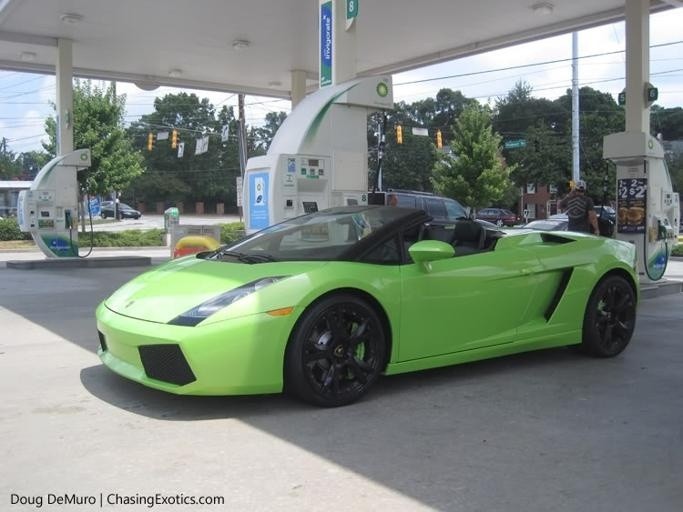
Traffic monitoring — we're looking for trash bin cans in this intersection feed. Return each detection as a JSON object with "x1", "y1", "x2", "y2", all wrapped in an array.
[{"x1": 164, "y1": 207, "x2": 179, "y2": 230}]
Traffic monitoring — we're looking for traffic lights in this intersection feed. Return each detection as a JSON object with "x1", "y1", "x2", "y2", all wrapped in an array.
[
  {"x1": 147, "y1": 133, "x2": 156, "y2": 152},
  {"x1": 168, "y1": 131, "x2": 172, "y2": 149},
  {"x1": 175, "y1": 130, "x2": 181, "y2": 150}
]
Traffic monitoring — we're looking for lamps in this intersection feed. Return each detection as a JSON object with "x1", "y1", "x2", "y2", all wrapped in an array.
[
  {"x1": 20, "y1": 12, "x2": 284, "y2": 89},
  {"x1": 529, "y1": 2, "x2": 555, "y2": 16}
]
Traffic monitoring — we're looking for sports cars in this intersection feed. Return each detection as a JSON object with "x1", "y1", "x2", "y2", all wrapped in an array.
[{"x1": 93, "y1": 201, "x2": 639, "y2": 407}]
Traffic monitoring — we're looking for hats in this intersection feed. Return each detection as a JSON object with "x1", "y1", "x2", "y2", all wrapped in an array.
[{"x1": 574, "y1": 179, "x2": 586, "y2": 191}]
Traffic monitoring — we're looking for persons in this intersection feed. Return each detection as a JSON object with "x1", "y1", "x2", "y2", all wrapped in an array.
[{"x1": 559, "y1": 179, "x2": 600, "y2": 236}]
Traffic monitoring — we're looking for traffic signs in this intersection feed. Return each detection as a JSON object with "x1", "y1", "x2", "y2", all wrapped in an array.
[
  {"x1": 156, "y1": 131, "x2": 169, "y2": 140},
  {"x1": 503, "y1": 139, "x2": 527, "y2": 149}
]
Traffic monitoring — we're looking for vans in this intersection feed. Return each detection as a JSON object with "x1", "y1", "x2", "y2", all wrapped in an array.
[{"x1": 367, "y1": 187, "x2": 472, "y2": 223}]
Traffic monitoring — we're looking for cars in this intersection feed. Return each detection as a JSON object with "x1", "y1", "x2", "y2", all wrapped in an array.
[
  {"x1": 97, "y1": 203, "x2": 142, "y2": 220},
  {"x1": 476, "y1": 205, "x2": 615, "y2": 237}
]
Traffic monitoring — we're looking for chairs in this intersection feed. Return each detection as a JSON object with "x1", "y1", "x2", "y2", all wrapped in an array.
[{"x1": 381, "y1": 220, "x2": 488, "y2": 265}]
